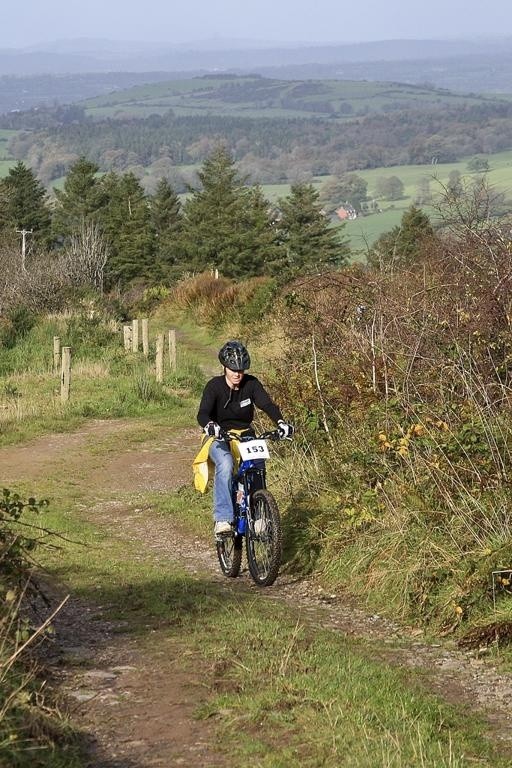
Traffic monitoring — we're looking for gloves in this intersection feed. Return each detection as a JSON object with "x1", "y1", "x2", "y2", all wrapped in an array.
[
  {"x1": 277, "y1": 418, "x2": 295, "y2": 440},
  {"x1": 203, "y1": 420, "x2": 223, "y2": 439}
]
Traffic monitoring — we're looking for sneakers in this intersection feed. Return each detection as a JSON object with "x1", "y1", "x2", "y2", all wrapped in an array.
[
  {"x1": 215, "y1": 521, "x2": 232, "y2": 533},
  {"x1": 254, "y1": 519, "x2": 272, "y2": 533}
]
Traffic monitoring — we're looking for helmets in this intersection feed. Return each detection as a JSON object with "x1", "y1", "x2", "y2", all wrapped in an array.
[{"x1": 218, "y1": 341, "x2": 250, "y2": 371}]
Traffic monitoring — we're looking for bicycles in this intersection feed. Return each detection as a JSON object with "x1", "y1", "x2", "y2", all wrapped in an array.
[{"x1": 203, "y1": 421, "x2": 294, "y2": 587}]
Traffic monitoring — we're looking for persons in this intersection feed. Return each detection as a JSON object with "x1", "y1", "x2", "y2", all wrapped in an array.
[{"x1": 195, "y1": 341, "x2": 297, "y2": 535}]
